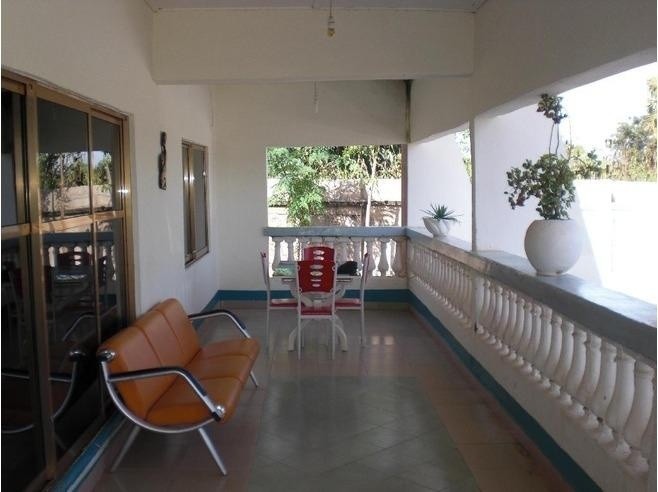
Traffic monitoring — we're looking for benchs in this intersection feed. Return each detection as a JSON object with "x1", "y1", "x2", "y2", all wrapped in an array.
[
  {"x1": 2, "y1": 304, "x2": 119, "y2": 454},
  {"x1": 97, "y1": 297, "x2": 260, "y2": 478}
]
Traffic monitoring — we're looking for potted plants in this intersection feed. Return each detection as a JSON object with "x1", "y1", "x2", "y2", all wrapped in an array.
[
  {"x1": 420, "y1": 203, "x2": 460, "y2": 239},
  {"x1": 504, "y1": 92, "x2": 584, "y2": 275}
]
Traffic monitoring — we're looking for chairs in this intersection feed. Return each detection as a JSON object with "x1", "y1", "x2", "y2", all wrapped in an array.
[
  {"x1": 2, "y1": 251, "x2": 114, "y2": 369},
  {"x1": 261, "y1": 246, "x2": 369, "y2": 361}
]
